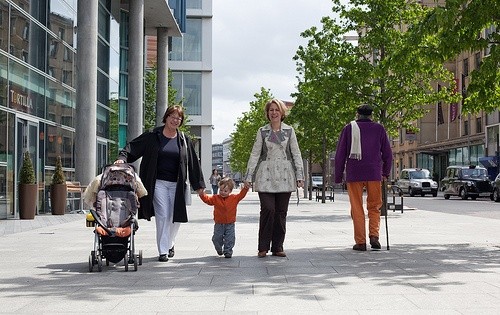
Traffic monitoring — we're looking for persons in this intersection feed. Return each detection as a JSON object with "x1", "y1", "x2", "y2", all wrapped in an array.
[
  {"x1": 334, "y1": 105, "x2": 392, "y2": 251},
  {"x1": 232, "y1": 170, "x2": 242, "y2": 188},
  {"x1": 209, "y1": 168, "x2": 222, "y2": 195},
  {"x1": 113, "y1": 104, "x2": 206, "y2": 262},
  {"x1": 245, "y1": 98, "x2": 306, "y2": 258},
  {"x1": 199, "y1": 184, "x2": 250, "y2": 258}
]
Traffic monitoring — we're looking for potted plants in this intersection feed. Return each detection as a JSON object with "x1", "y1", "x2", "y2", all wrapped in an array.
[
  {"x1": 16, "y1": 150, "x2": 38, "y2": 219},
  {"x1": 50, "y1": 155, "x2": 67, "y2": 215}
]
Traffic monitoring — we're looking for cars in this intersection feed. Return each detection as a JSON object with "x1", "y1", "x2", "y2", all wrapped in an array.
[
  {"x1": 490, "y1": 172, "x2": 500, "y2": 202},
  {"x1": 312, "y1": 176, "x2": 327, "y2": 191},
  {"x1": 440, "y1": 163, "x2": 494, "y2": 200},
  {"x1": 395, "y1": 167, "x2": 438, "y2": 197}
]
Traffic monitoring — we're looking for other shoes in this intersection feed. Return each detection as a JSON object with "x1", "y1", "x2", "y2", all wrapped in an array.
[
  {"x1": 352, "y1": 243, "x2": 367, "y2": 251},
  {"x1": 225, "y1": 254, "x2": 231, "y2": 258},
  {"x1": 258, "y1": 250, "x2": 266, "y2": 257},
  {"x1": 168, "y1": 246, "x2": 174, "y2": 257},
  {"x1": 272, "y1": 251, "x2": 287, "y2": 257},
  {"x1": 159, "y1": 254, "x2": 169, "y2": 262},
  {"x1": 369, "y1": 236, "x2": 381, "y2": 249}
]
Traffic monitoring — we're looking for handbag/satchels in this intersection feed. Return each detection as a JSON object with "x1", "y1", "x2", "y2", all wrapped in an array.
[{"x1": 185, "y1": 180, "x2": 192, "y2": 206}]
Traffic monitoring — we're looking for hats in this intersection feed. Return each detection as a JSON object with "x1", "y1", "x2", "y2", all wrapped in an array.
[{"x1": 357, "y1": 103, "x2": 373, "y2": 115}]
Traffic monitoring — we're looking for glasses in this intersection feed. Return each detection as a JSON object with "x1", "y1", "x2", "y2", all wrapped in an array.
[{"x1": 171, "y1": 115, "x2": 183, "y2": 121}]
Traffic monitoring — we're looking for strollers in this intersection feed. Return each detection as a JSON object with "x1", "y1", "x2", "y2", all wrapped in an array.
[{"x1": 85, "y1": 164, "x2": 142, "y2": 272}]
